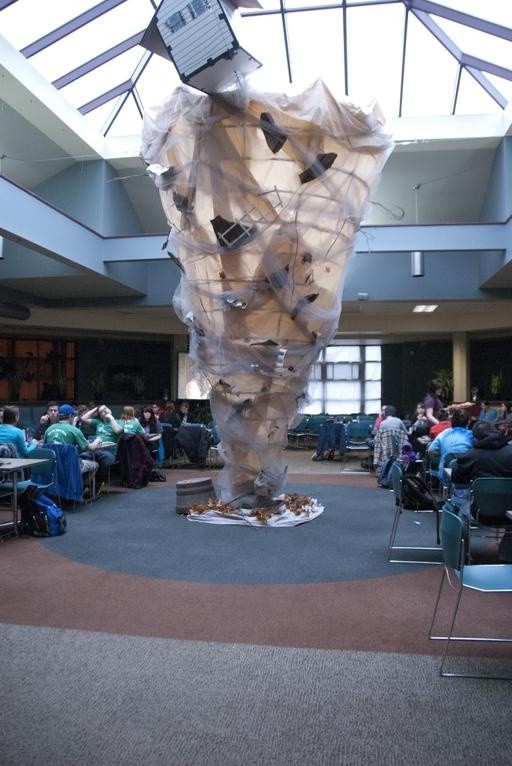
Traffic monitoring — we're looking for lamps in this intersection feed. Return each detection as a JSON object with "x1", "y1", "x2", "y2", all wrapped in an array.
[{"x1": 410, "y1": 182, "x2": 425, "y2": 278}]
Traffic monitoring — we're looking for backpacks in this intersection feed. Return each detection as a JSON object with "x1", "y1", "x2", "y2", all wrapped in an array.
[
  {"x1": 398, "y1": 476, "x2": 445, "y2": 511},
  {"x1": 378, "y1": 456, "x2": 402, "y2": 488},
  {"x1": 20, "y1": 485, "x2": 66, "y2": 537}
]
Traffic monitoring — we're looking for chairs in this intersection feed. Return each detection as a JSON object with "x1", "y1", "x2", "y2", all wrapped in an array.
[
  {"x1": 0, "y1": 405, "x2": 220, "y2": 546},
  {"x1": 427, "y1": 504, "x2": 512, "y2": 682},
  {"x1": 287, "y1": 413, "x2": 512, "y2": 565}
]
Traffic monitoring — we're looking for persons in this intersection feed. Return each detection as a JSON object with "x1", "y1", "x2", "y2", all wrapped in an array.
[
  {"x1": 1, "y1": 399, "x2": 193, "y2": 506},
  {"x1": 363, "y1": 378, "x2": 512, "y2": 566},
  {"x1": 15, "y1": 350, "x2": 42, "y2": 402},
  {"x1": 40, "y1": 349, "x2": 65, "y2": 401}
]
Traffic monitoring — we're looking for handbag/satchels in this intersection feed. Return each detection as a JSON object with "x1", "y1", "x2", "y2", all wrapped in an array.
[
  {"x1": 150, "y1": 470, "x2": 167, "y2": 482},
  {"x1": 310, "y1": 447, "x2": 334, "y2": 460}
]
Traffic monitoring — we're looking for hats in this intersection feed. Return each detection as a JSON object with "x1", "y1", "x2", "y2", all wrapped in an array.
[{"x1": 59, "y1": 405, "x2": 74, "y2": 416}]
